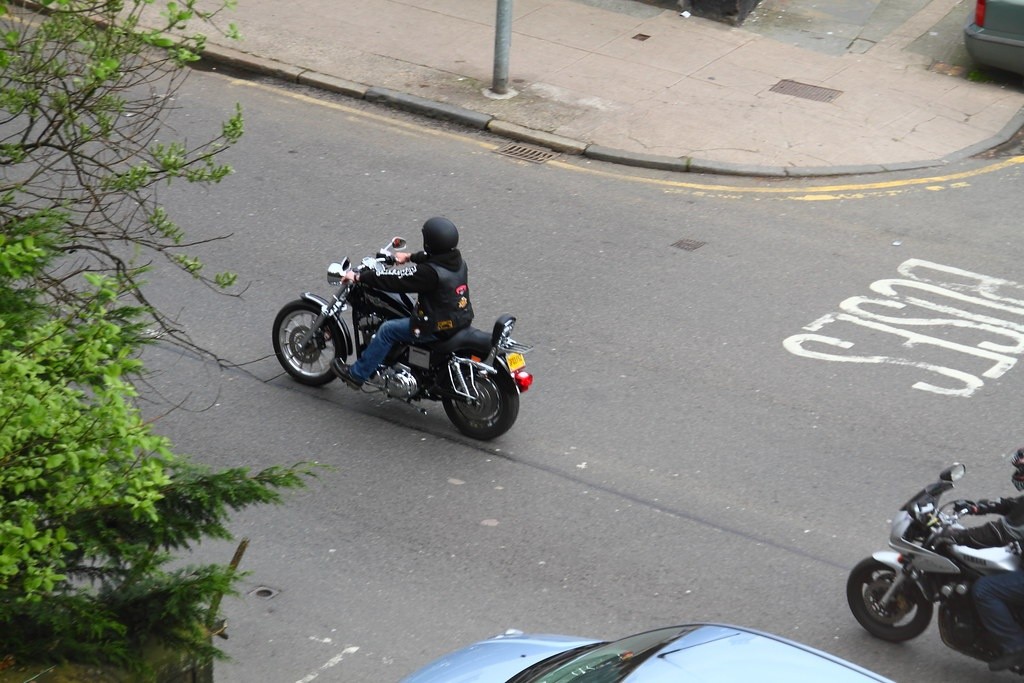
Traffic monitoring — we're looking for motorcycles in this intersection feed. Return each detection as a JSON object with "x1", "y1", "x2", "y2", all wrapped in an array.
[
  {"x1": 846, "y1": 462, "x2": 1023, "y2": 675},
  {"x1": 271, "y1": 235, "x2": 533, "y2": 441}
]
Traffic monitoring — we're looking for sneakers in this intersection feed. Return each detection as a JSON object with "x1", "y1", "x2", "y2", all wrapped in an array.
[{"x1": 989, "y1": 649, "x2": 1024, "y2": 672}]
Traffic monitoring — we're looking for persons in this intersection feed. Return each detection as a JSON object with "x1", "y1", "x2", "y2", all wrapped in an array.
[
  {"x1": 330, "y1": 216, "x2": 474, "y2": 390},
  {"x1": 926, "y1": 448, "x2": 1024, "y2": 671}
]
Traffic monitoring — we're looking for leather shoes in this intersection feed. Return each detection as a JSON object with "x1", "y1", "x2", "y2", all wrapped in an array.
[{"x1": 330, "y1": 357, "x2": 364, "y2": 391}]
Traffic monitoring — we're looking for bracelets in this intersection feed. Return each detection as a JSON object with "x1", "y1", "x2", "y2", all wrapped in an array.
[{"x1": 354, "y1": 273, "x2": 358, "y2": 282}]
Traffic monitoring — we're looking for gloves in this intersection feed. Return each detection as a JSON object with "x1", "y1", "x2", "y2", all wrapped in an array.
[
  {"x1": 934, "y1": 529, "x2": 963, "y2": 549},
  {"x1": 954, "y1": 498, "x2": 988, "y2": 516}
]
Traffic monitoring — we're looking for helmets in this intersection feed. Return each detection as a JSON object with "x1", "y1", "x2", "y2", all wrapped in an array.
[
  {"x1": 421, "y1": 217, "x2": 459, "y2": 254},
  {"x1": 1010, "y1": 447, "x2": 1024, "y2": 492}
]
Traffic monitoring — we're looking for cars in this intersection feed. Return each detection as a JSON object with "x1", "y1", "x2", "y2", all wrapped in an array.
[{"x1": 399, "y1": 622, "x2": 894, "y2": 683}]
